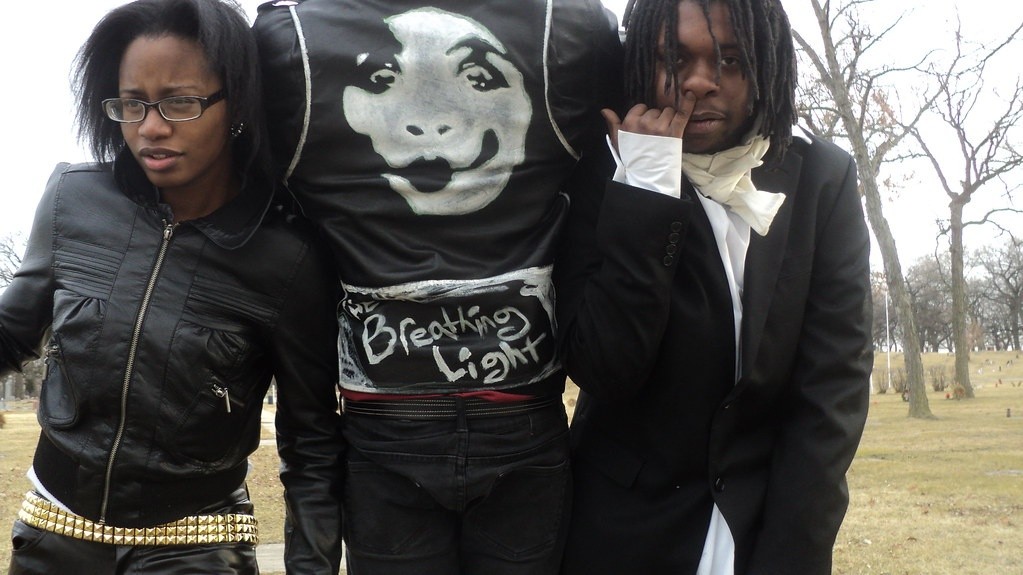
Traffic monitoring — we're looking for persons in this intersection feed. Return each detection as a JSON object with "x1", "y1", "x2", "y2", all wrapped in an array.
[
  {"x1": 0, "y1": 0, "x2": 343, "y2": 575},
  {"x1": 249, "y1": 0, "x2": 623, "y2": 575},
  {"x1": 554, "y1": 1, "x2": 875, "y2": 575}
]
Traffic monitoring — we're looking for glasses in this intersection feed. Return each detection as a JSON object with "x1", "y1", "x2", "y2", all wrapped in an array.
[{"x1": 102, "y1": 88, "x2": 227, "y2": 123}]
